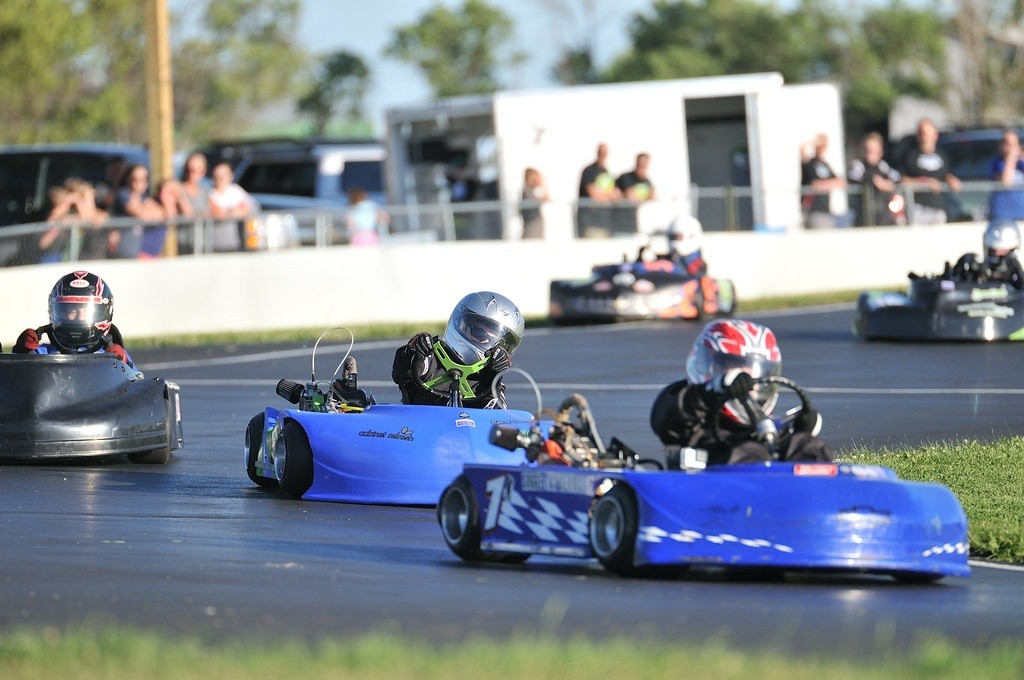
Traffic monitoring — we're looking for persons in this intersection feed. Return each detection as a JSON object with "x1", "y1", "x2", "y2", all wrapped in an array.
[
  {"x1": 988, "y1": 130, "x2": 1024, "y2": 220},
  {"x1": 666, "y1": 319, "x2": 832, "y2": 464},
  {"x1": 657, "y1": 215, "x2": 706, "y2": 279},
  {"x1": 801, "y1": 134, "x2": 846, "y2": 228},
  {"x1": 847, "y1": 131, "x2": 902, "y2": 226},
  {"x1": 38, "y1": 150, "x2": 257, "y2": 263},
  {"x1": 902, "y1": 120, "x2": 972, "y2": 222},
  {"x1": 577, "y1": 143, "x2": 656, "y2": 239},
  {"x1": 391, "y1": 292, "x2": 525, "y2": 410},
  {"x1": 952, "y1": 223, "x2": 1024, "y2": 291},
  {"x1": 13, "y1": 271, "x2": 137, "y2": 372},
  {"x1": 345, "y1": 187, "x2": 390, "y2": 245},
  {"x1": 522, "y1": 168, "x2": 550, "y2": 239}
]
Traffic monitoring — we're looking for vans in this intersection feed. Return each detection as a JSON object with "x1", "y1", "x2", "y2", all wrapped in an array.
[
  {"x1": 0, "y1": 141, "x2": 157, "y2": 229},
  {"x1": 880, "y1": 129, "x2": 1023, "y2": 182},
  {"x1": 231, "y1": 144, "x2": 386, "y2": 210}
]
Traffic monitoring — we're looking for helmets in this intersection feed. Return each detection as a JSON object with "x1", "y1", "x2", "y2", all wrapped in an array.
[
  {"x1": 685, "y1": 318, "x2": 782, "y2": 428},
  {"x1": 48, "y1": 271, "x2": 114, "y2": 347},
  {"x1": 982, "y1": 219, "x2": 1020, "y2": 264},
  {"x1": 443, "y1": 291, "x2": 525, "y2": 366},
  {"x1": 668, "y1": 216, "x2": 702, "y2": 257}
]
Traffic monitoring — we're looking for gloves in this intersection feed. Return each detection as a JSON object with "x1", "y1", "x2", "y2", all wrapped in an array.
[
  {"x1": 699, "y1": 368, "x2": 755, "y2": 411},
  {"x1": 794, "y1": 410, "x2": 824, "y2": 437},
  {"x1": 404, "y1": 332, "x2": 434, "y2": 360},
  {"x1": 16, "y1": 329, "x2": 42, "y2": 351},
  {"x1": 484, "y1": 345, "x2": 509, "y2": 373},
  {"x1": 106, "y1": 344, "x2": 127, "y2": 365}
]
